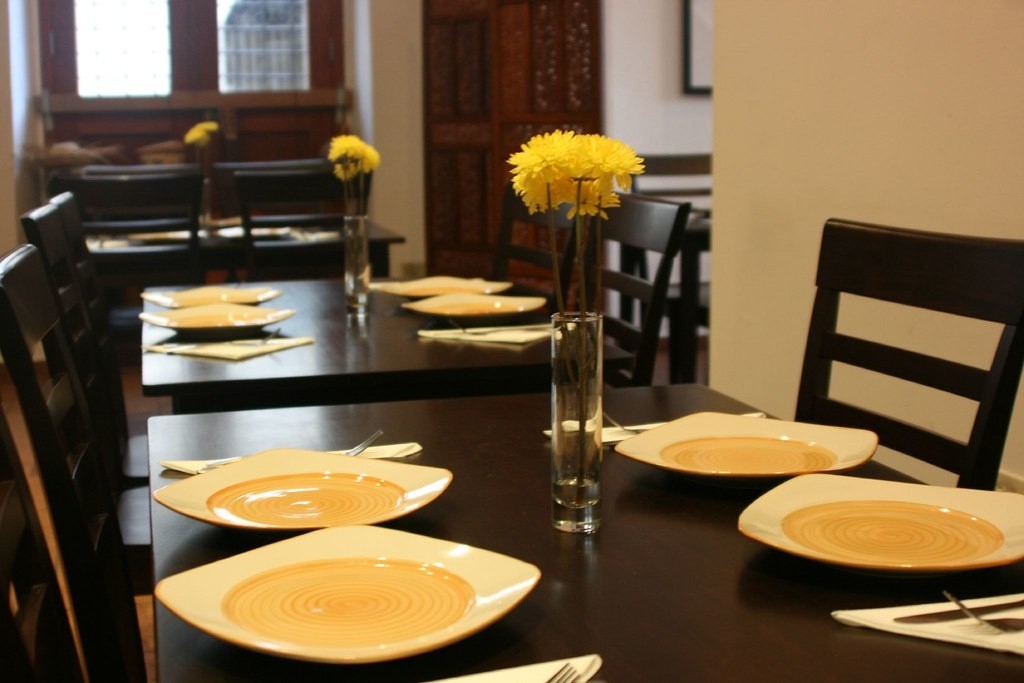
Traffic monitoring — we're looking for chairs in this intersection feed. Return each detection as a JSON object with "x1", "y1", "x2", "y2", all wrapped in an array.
[
  {"x1": 0, "y1": 160, "x2": 201, "y2": 683},
  {"x1": 793, "y1": 217, "x2": 1024, "y2": 492},
  {"x1": 211, "y1": 155, "x2": 372, "y2": 226},
  {"x1": 489, "y1": 184, "x2": 578, "y2": 316},
  {"x1": 580, "y1": 191, "x2": 689, "y2": 386},
  {"x1": 617, "y1": 155, "x2": 711, "y2": 385}
]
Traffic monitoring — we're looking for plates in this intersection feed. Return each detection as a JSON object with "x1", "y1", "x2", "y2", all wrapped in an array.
[
  {"x1": 137, "y1": 305, "x2": 294, "y2": 336},
  {"x1": 152, "y1": 449, "x2": 451, "y2": 529},
  {"x1": 400, "y1": 290, "x2": 546, "y2": 323},
  {"x1": 617, "y1": 414, "x2": 879, "y2": 477},
  {"x1": 738, "y1": 472, "x2": 1024, "y2": 569},
  {"x1": 368, "y1": 272, "x2": 513, "y2": 298},
  {"x1": 139, "y1": 284, "x2": 281, "y2": 309},
  {"x1": 152, "y1": 526, "x2": 540, "y2": 662}
]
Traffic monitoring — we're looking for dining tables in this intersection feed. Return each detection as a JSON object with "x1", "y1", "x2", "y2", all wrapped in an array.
[
  {"x1": 142, "y1": 277, "x2": 635, "y2": 412},
  {"x1": 144, "y1": 381, "x2": 1024, "y2": 683}
]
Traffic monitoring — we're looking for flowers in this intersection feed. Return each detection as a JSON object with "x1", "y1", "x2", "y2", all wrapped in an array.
[
  {"x1": 184, "y1": 121, "x2": 221, "y2": 171},
  {"x1": 329, "y1": 135, "x2": 379, "y2": 216},
  {"x1": 504, "y1": 130, "x2": 645, "y2": 316}
]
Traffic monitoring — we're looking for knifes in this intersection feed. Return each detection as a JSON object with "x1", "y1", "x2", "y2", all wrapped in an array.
[{"x1": 892, "y1": 594, "x2": 1023, "y2": 623}]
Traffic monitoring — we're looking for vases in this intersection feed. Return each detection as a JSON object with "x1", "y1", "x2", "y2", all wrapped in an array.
[
  {"x1": 194, "y1": 153, "x2": 211, "y2": 186},
  {"x1": 549, "y1": 310, "x2": 604, "y2": 535},
  {"x1": 342, "y1": 217, "x2": 371, "y2": 318}
]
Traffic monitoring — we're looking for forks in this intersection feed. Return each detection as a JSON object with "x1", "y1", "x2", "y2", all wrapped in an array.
[
  {"x1": 944, "y1": 591, "x2": 1024, "y2": 632},
  {"x1": 602, "y1": 408, "x2": 657, "y2": 433},
  {"x1": 544, "y1": 663, "x2": 580, "y2": 683}
]
